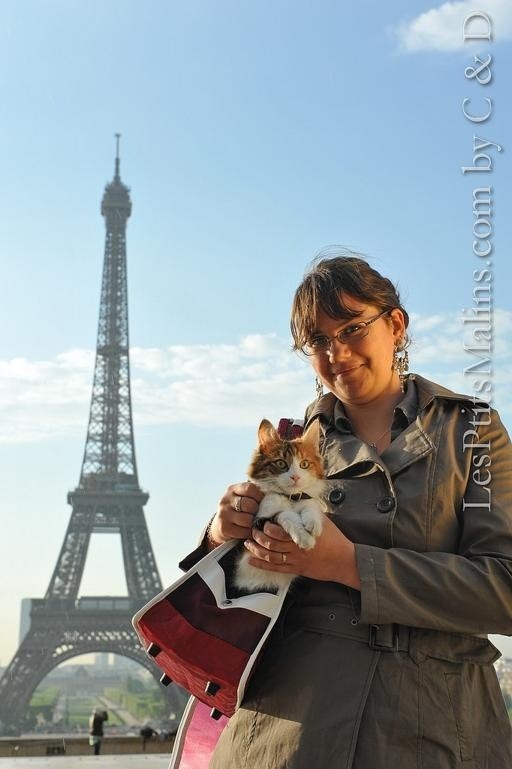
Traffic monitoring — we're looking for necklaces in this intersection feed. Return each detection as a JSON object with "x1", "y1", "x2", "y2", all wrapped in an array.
[{"x1": 353, "y1": 428, "x2": 389, "y2": 455}]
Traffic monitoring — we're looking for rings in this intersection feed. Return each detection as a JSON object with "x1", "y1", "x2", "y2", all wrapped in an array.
[
  {"x1": 233, "y1": 495, "x2": 243, "y2": 512},
  {"x1": 281, "y1": 553, "x2": 288, "y2": 564}
]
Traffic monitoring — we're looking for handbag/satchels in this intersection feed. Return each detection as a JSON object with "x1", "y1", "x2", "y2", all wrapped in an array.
[{"x1": 130, "y1": 418, "x2": 305, "y2": 721}]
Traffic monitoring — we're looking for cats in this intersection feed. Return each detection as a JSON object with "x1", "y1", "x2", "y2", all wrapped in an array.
[{"x1": 230, "y1": 416, "x2": 328, "y2": 597}]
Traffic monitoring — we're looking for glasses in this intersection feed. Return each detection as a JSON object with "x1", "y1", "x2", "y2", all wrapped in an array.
[{"x1": 300, "y1": 310, "x2": 391, "y2": 358}]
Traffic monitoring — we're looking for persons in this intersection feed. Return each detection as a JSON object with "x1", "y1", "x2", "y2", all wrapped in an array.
[
  {"x1": 164, "y1": 254, "x2": 510, "y2": 768},
  {"x1": 89, "y1": 705, "x2": 109, "y2": 754},
  {"x1": 139, "y1": 721, "x2": 158, "y2": 753}
]
